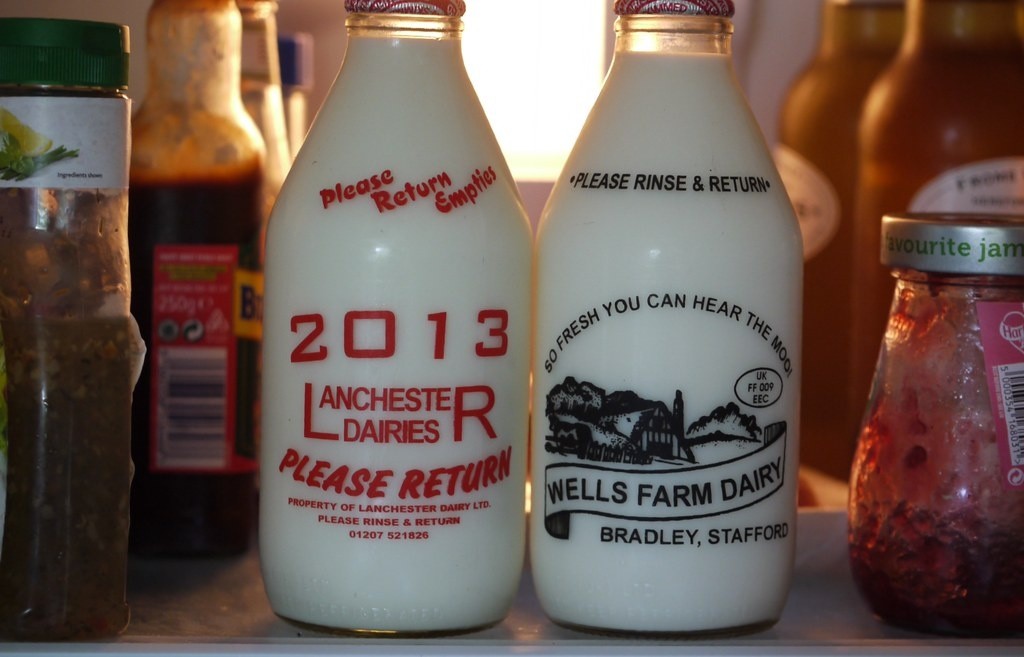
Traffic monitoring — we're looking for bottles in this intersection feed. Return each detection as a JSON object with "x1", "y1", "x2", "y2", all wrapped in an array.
[{"x1": 0, "y1": 2, "x2": 1024, "y2": 643}]
